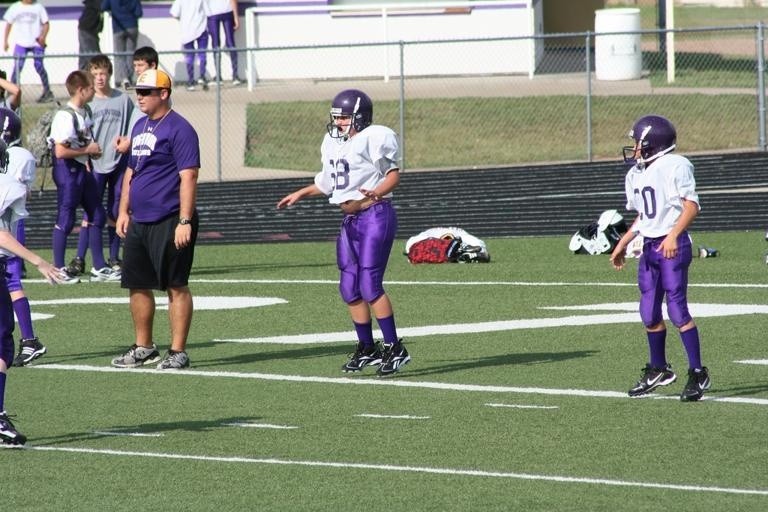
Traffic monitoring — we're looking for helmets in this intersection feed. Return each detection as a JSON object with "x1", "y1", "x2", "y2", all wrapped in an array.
[
  {"x1": 0, "y1": 139, "x2": 9, "y2": 174},
  {"x1": 326, "y1": 89, "x2": 374, "y2": 141},
  {"x1": 0, "y1": 107, "x2": 22, "y2": 148},
  {"x1": 621, "y1": 115, "x2": 677, "y2": 172}
]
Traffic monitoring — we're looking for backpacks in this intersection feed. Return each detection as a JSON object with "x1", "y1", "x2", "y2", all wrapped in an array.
[{"x1": 26, "y1": 104, "x2": 93, "y2": 169}]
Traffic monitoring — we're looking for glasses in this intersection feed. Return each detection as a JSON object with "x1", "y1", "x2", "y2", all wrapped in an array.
[{"x1": 135, "y1": 89, "x2": 151, "y2": 95}]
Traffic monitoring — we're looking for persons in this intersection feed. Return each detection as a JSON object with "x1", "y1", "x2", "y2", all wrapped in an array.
[
  {"x1": 113, "y1": 45, "x2": 173, "y2": 155},
  {"x1": 203, "y1": 1, "x2": 242, "y2": 87},
  {"x1": 611, "y1": 114, "x2": 714, "y2": 402},
  {"x1": 100, "y1": 1, "x2": 142, "y2": 88},
  {"x1": 0, "y1": 70, "x2": 45, "y2": 448},
  {"x1": 276, "y1": 89, "x2": 412, "y2": 377},
  {"x1": 2, "y1": 1, "x2": 56, "y2": 103},
  {"x1": 110, "y1": 69, "x2": 202, "y2": 372},
  {"x1": 78, "y1": 0, "x2": 103, "y2": 70},
  {"x1": 169, "y1": 0, "x2": 213, "y2": 93},
  {"x1": 47, "y1": 72, "x2": 122, "y2": 286},
  {"x1": 70, "y1": 52, "x2": 134, "y2": 271}
]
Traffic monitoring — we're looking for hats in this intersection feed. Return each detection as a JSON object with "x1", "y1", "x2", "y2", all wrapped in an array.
[{"x1": 126, "y1": 68, "x2": 171, "y2": 90}]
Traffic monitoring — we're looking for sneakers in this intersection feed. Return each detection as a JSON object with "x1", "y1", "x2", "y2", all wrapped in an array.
[
  {"x1": 455, "y1": 242, "x2": 481, "y2": 254},
  {"x1": 628, "y1": 362, "x2": 677, "y2": 398},
  {"x1": 52, "y1": 257, "x2": 122, "y2": 284},
  {"x1": 699, "y1": 247, "x2": 720, "y2": 259},
  {"x1": 341, "y1": 341, "x2": 382, "y2": 373},
  {"x1": 1, "y1": 412, "x2": 27, "y2": 450},
  {"x1": 375, "y1": 338, "x2": 411, "y2": 378},
  {"x1": 680, "y1": 366, "x2": 712, "y2": 402},
  {"x1": 185, "y1": 75, "x2": 241, "y2": 90},
  {"x1": 111, "y1": 342, "x2": 161, "y2": 368},
  {"x1": 11, "y1": 336, "x2": 47, "y2": 367},
  {"x1": 457, "y1": 252, "x2": 490, "y2": 264},
  {"x1": 156, "y1": 349, "x2": 192, "y2": 372},
  {"x1": 37, "y1": 91, "x2": 54, "y2": 103}
]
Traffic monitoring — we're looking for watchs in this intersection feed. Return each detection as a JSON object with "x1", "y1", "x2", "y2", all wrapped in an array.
[{"x1": 177, "y1": 219, "x2": 192, "y2": 225}]
